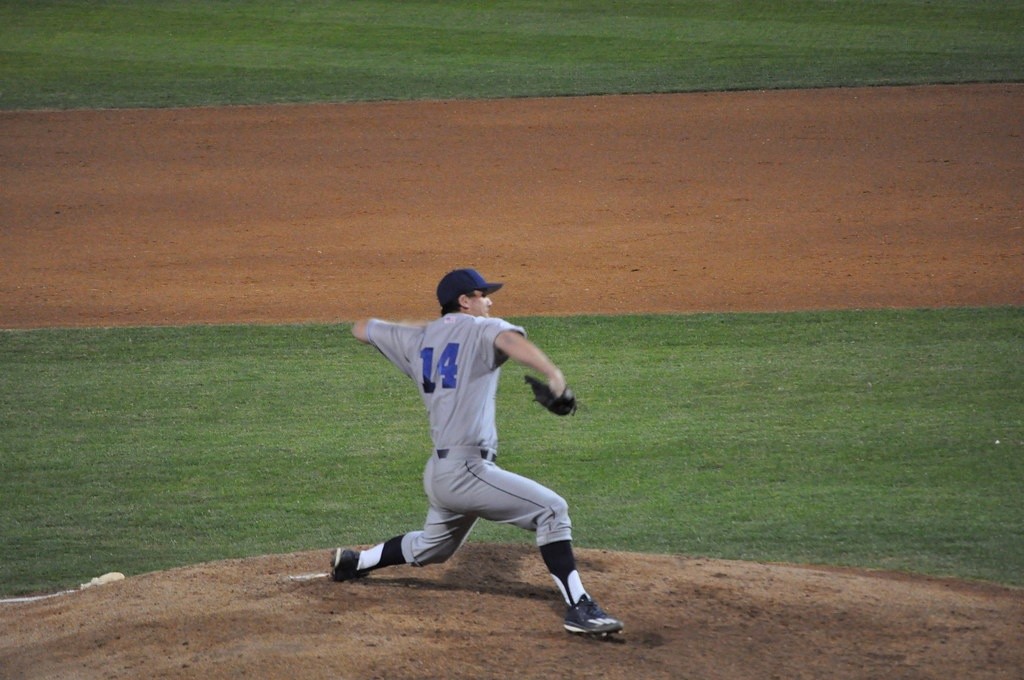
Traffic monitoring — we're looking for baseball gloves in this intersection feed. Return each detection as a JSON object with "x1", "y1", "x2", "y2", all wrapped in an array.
[{"x1": 521, "y1": 374, "x2": 578, "y2": 417}]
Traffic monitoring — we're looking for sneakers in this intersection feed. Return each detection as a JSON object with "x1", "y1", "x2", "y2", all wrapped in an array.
[
  {"x1": 329, "y1": 547, "x2": 369, "y2": 582},
  {"x1": 563, "y1": 594, "x2": 624, "y2": 637}
]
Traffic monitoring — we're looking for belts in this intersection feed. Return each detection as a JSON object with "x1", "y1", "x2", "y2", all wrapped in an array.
[{"x1": 437, "y1": 449, "x2": 497, "y2": 462}]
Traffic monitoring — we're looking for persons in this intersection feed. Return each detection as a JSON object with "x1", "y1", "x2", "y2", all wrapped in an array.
[{"x1": 329, "y1": 265, "x2": 624, "y2": 638}]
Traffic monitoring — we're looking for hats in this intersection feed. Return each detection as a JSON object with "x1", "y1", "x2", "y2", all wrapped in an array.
[{"x1": 437, "y1": 269, "x2": 504, "y2": 306}]
